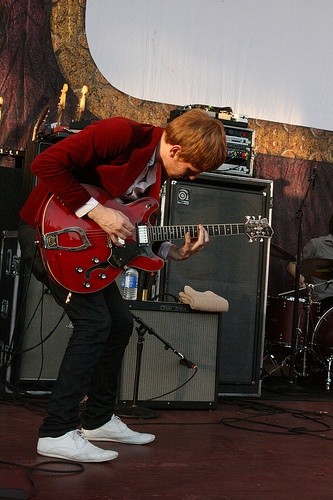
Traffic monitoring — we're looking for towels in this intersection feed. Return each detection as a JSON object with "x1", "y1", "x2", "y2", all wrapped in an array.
[{"x1": 179, "y1": 285, "x2": 228, "y2": 312}]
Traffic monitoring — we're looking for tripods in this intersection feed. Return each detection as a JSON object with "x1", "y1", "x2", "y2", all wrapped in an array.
[{"x1": 263, "y1": 169, "x2": 333, "y2": 401}]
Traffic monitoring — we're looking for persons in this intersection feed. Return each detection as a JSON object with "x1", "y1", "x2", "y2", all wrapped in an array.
[
  {"x1": 287, "y1": 213, "x2": 333, "y2": 387},
  {"x1": 16, "y1": 109, "x2": 227, "y2": 463}
]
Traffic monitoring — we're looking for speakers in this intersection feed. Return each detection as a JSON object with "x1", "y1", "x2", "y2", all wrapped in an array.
[
  {"x1": 116, "y1": 300, "x2": 221, "y2": 409},
  {"x1": 5, "y1": 238, "x2": 123, "y2": 395},
  {"x1": 155, "y1": 171, "x2": 274, "y2": 399}
]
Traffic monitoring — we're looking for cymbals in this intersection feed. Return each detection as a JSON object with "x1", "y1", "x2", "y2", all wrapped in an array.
[
  {"x1": 298, "y1": 257, "x2": 333, "y2": 279},
  {"x1": 270, "y1": 239, "x2": 298, "y2": 263}
]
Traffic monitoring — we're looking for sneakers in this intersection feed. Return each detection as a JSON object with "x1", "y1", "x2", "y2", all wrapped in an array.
[
  {"x1": 81, "y1": 413, "x2": 155, "y2": 445},
  {"x1": 37, "y1": 428, "x2": 118, "y2": 463}
]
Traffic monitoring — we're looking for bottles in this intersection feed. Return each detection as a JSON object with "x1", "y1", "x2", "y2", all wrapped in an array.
[{"x1": 122, "y1": 268, "x2": 138, "y2": 300}]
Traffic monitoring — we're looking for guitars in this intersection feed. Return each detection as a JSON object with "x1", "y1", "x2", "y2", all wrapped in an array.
[{"x1": 39, "y1": 181, "x2": 273, "y2": 294}]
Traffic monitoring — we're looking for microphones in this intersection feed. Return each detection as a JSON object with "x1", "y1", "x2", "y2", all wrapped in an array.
[
  {"x1": 312, "y1": 159, "x2": 317, "y2": 190},
  {"x1": 180, "y1": 359, "x2": 198, "y2": 370}
]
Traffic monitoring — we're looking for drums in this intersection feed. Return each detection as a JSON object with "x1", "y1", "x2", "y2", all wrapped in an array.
[
  {"x1": 265, "y1": 295, "x2": 316, "y2": 351},
  {"x1": 309, "y1": 297, "x2": 333, "y2": 371}
]
